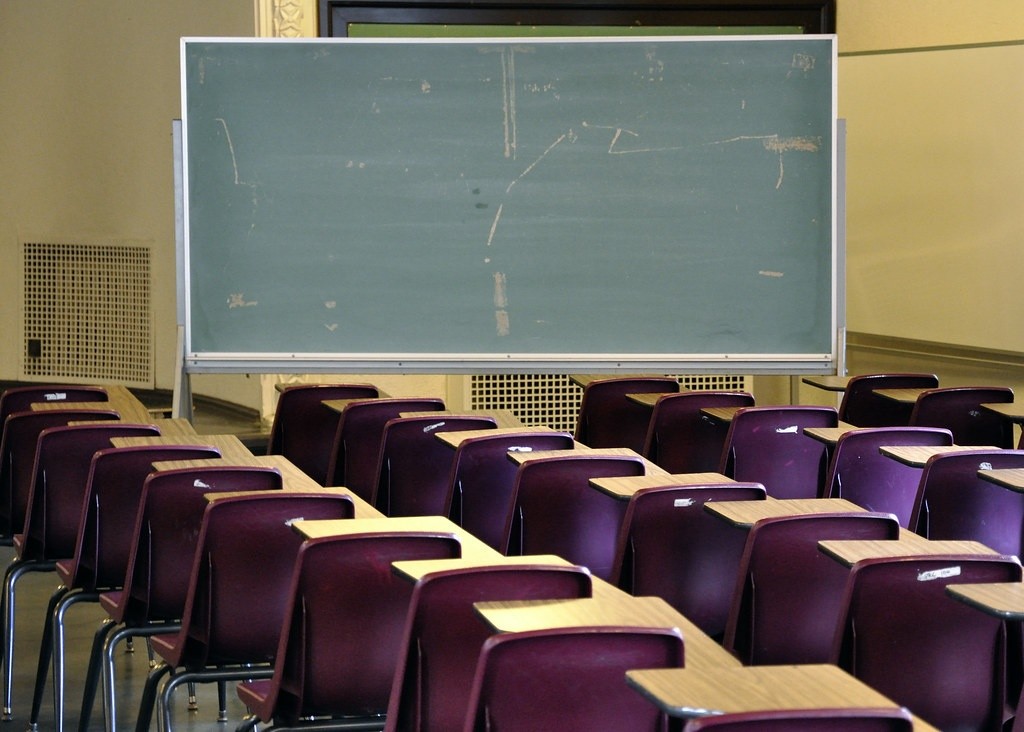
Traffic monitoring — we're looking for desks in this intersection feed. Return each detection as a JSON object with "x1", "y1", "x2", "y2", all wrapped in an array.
[{"x1": 29, "y1": 372, "x2": 1024, "y2": 732}]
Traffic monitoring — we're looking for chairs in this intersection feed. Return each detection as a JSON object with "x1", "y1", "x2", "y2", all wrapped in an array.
[
  {"x1": 906, "y1": 449, "x2": 1024, "y2": 566},
  {"x1": 461, "y1": 625, "x2": 686, "y2": 732},
  {"x1": 606, "y1": 480, "x2": 768, "y2": 643},
  {"x1": 382, "y1": 564, "x2": 593, "y2": 732},
  {"x1": 641, "y1": 391, "x2": 756, "y2": 475},
  {"x1": 0, "y1": 378, "x2": 500, "y2": 732},
  {"x1": 502, "y1": 455, "x2": 646, "y2": 584},
  {"x1": 441, "y1": 430, "x2": 576, "y2": 556},
  {"x1": 823, "y1": 427, "x2": 955, "y2": 533},
  {"x1": 838, "y1": 373, "x2": 939, "y2": 429},
  {"x1": 720, "y1": 510, "x2": 901, "y2": 665},
  {"x1": 828, "y1": 554, "x2": 1024, "y2": 732},
  {"x1": 573, "y1": 377, "x2": 681, "y2": 455},
  {"x1": 910, "y1": 385, "x2": 1015, "y2": 451},
  {"x1": 718, "y1": 406, "x2": 839, "y2": 499}
]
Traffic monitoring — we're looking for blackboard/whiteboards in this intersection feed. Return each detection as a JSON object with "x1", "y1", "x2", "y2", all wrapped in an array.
[{"x1": 178, "y1": 33, "x2": 836, "y2": 365}]
